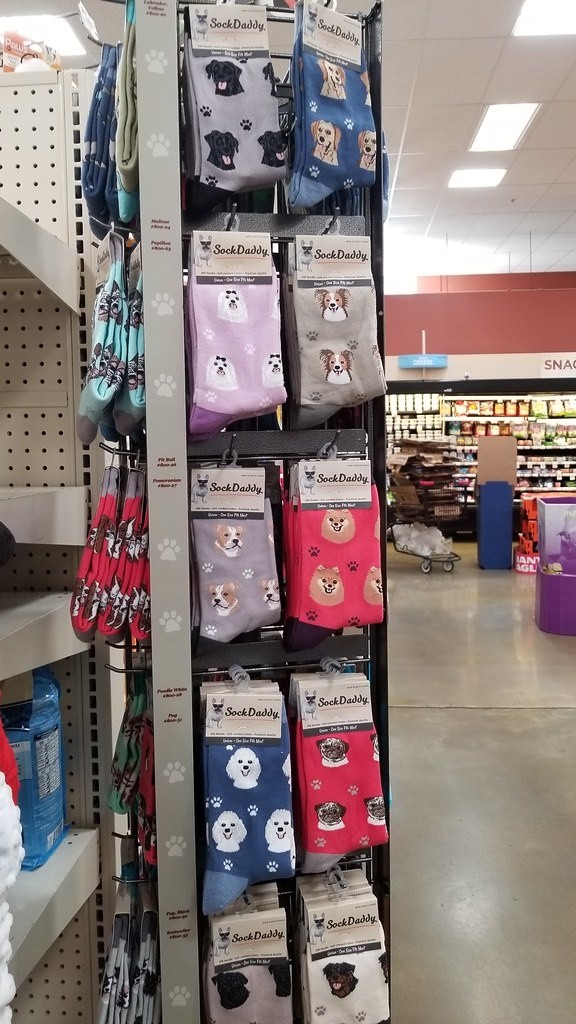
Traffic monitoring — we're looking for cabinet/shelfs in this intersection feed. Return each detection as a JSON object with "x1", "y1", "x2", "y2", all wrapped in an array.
[
  {"x1": 0, "y1": 0, "x2": 108, "y2": 1024},
  {"x1": 437, "y1": 392, "x2": 576, "y2": 539}
]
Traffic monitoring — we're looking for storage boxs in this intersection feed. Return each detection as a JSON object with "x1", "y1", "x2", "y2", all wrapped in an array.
[{"x1": 471, "y1": 437, "x2": 576, "y2": 637}]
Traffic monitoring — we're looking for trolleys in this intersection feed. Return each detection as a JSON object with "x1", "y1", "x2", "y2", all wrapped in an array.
[
  {"x1": 418, "y1": 479, "x2": 471, "y2": 538},
  {"x1": 385, "y1": 485, "x2": 462, "y2": 574}
]
